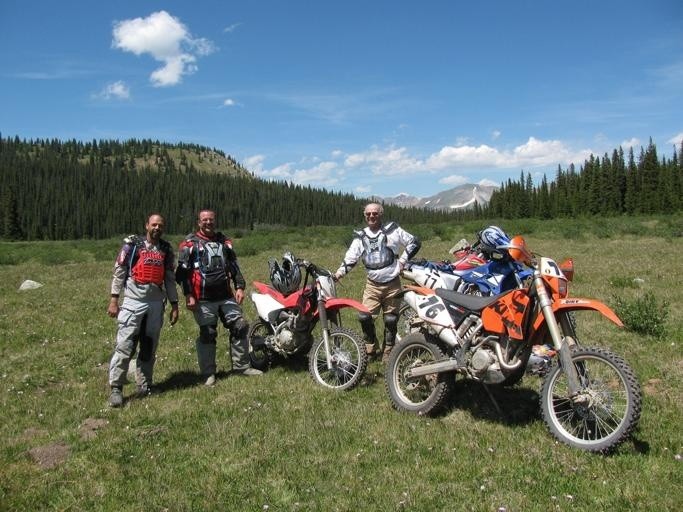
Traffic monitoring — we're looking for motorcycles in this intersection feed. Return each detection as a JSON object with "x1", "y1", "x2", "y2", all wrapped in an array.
[{"x1": 247, "y1": 258, "x2": 373, "y2": 392}]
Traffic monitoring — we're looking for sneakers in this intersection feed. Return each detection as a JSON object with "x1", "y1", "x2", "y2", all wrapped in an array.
[
  {"x1": 380, "y1": 351, "x2": 391, "y2": 363},
  {"x1": 136, "y1": 383, "x2": 152, "y2": 398},
  {"x1": 108, "y1": 385, "x2": 124, "y2": 408},
  {"x1": 365, "y1": 349, "x2": 378, "y2": 364},
  {"x1": 231, "y1": 367, "x2": 264, "y2": 376},
  {"x1": 202, "y1": 370, "x2": 215, "y2": 386}
]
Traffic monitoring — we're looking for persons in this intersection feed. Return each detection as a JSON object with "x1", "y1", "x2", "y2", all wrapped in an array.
[
  {"x1": 107, "y1": 213, "x2": 179, "y2": 409},
  {"x1": 176, "y1": 209, "x2": 264, "y2": 386},
  {"x1": 333, "y1": 203, "x2": 421, "y2": 365}
]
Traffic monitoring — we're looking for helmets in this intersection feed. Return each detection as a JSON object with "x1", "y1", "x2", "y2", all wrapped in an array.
[
  {"x1": 267, "y1": 251, "x2": 302, "y2": 296},
  {"x1": 477, "y1": 224, "x2": 509, "y2": 254}
]
